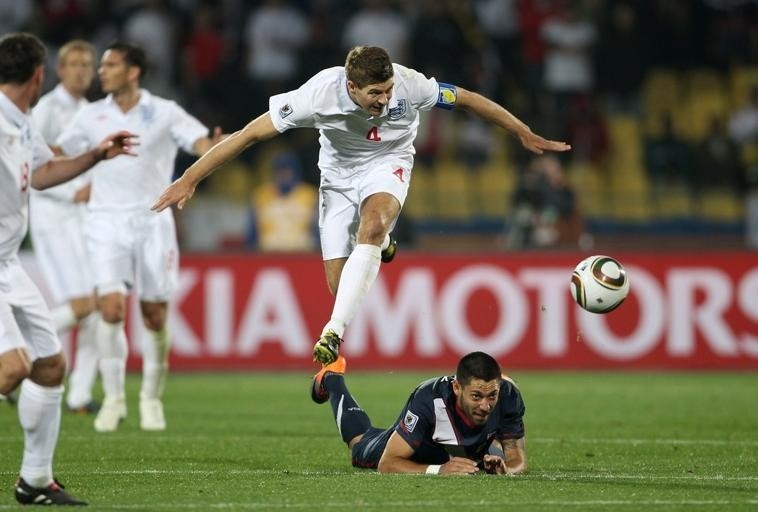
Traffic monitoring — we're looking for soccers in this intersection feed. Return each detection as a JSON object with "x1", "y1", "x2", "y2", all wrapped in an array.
[{"x1": 570, "y1": 254, "x2": 631, "y2": 314}]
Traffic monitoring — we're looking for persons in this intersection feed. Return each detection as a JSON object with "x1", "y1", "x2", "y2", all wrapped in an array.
[
  {"x1": 46, "y1": 43, "x2": 232, "y2": 433},
  {"x1": 310, "y1": 350, "x2": 529, "y2": 477},
  {"x1": 0, "y1": 34, "x2": 139, "y2": 508},
  {"x1": 149, "y1": 42, "x2": 571, "y2": 365},
  {"x1": 28, "y1": 40, "x2": 97, "y2": 415}
]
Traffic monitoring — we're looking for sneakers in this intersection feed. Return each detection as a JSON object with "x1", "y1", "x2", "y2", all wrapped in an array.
[
  {"x1": 312, "y1": 329, "x2": 345, "y2": 365},
  {"x1": 139, "y1": 398, "x2": 167, "y2": 430},
  {"x1": 311, "y1": 355, "x2": 346, "y2": 405},
  {"x1": 15, "y1": 476, "x2": 88, "y2": 506},
  {"x1": 93, "y1": 393, "x2": 127, "y2": 433},
  {"x1": 380, "y1": 233, "x2": 398, "y2": 263},
  {"x1": 63, "y1": 400, "x2": 101, "y2": 416}
]
